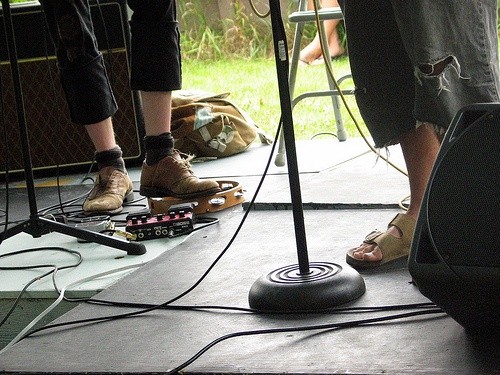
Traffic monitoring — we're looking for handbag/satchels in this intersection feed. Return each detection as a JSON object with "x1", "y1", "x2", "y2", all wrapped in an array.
[{"x1": 167, "y1": 91, "x2": 258, "y2": 159}]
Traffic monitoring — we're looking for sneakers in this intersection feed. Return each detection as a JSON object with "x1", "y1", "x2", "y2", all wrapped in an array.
[
  {"x1": 82, "y1": 166, "x2": 134, "y2": 214},
  {"x1": 139, "y1": 152, "x2": 222, "y2": 195}
]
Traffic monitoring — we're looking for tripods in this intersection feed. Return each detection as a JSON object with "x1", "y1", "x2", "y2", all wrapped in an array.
[{"x1": 0, "y1": 0, "x2": 147, "y2": 256}]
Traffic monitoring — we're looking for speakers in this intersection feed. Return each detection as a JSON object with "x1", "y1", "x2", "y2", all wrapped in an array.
[
  {"x1": 409, "y1": 101, "x2": 500, "y2": 329},
  {"x1": 0, "y1": 0, "x2": 148, "y2": 180}
]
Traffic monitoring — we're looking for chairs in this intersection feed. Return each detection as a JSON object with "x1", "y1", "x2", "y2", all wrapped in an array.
[{"x1": 274, "y1": 0, "x2": 356, "y2": 166}]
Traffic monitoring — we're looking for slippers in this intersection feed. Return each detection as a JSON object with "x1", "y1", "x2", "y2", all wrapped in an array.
[
  {"x1": 314, "y1": 56, "x2": 337, "y2": 67},
  {"x1": 290, "y1": 55, "x2": 312, "y2": 68}
]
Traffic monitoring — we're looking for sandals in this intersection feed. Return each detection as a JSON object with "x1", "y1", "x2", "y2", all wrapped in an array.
[{"x1": 346, "y1": 213, "x2": 428, "y2": 270}]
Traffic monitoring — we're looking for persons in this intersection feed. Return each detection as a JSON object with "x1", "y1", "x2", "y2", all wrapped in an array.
[
  {"x1": 336, "y1": 0, "x2": 500, "y2": 274},
  {"x1": 37, "y1": 0, "x2": 222, "y2": 216},
  {"x1": 298, "y1": 0, "x2": 345, "y2": 65}
]
